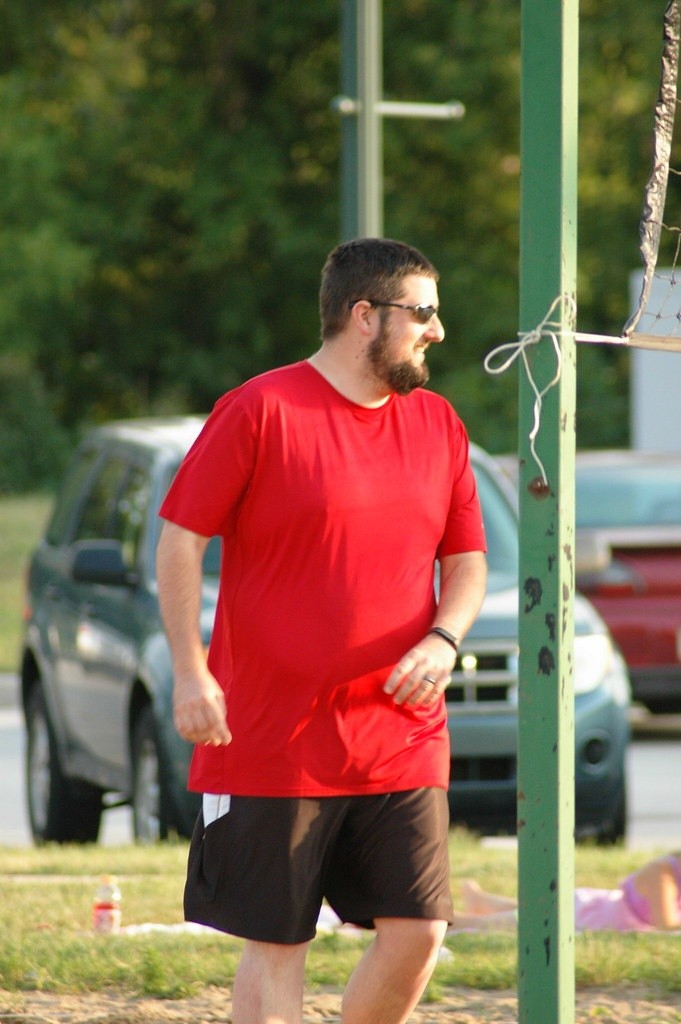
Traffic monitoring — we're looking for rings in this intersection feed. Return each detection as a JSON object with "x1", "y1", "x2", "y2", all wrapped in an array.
[{"x1": 424, "y1": 676, "x2": 435, "y2": 684}]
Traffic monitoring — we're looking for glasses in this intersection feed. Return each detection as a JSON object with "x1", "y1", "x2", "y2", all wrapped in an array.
[{"x1": 349, "y1": 297, "x2": 441, "y2": 325}]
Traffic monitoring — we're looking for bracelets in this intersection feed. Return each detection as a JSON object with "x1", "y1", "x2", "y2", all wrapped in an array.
[{"x1": 428, "y1": 627, "x2": 459, "y2": 651}]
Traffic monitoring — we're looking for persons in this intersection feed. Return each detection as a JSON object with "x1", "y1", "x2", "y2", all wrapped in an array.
[
  {"x1": 448, "y1": 851, "x2": 681, "y2": 929},
  {"x1": 155, "y1": 236, "x2": 488, "y2": 1023}
]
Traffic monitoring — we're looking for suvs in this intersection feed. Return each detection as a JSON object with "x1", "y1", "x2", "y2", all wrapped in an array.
[{"x1": 19, "y1": 411, "x2": 638, "y2": 848}]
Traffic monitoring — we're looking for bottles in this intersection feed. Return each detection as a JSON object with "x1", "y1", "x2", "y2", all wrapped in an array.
[{"x1": 91, "y1": 874, "x2": 122, "y2": 935}]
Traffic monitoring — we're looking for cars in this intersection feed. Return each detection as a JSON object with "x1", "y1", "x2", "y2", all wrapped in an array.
[{"x1": 494, "y1": 449, "x2": 681, "y2": 718}]
